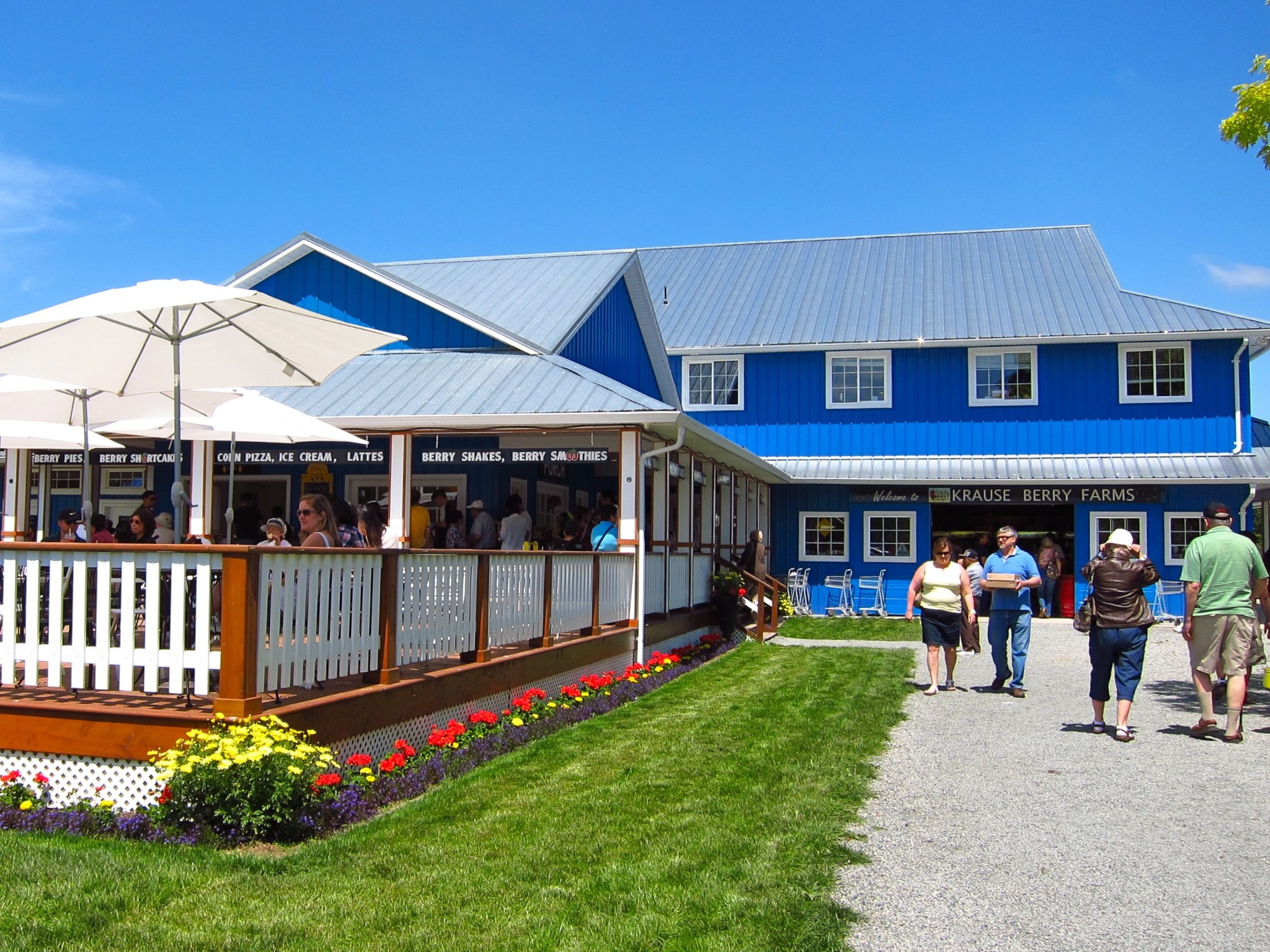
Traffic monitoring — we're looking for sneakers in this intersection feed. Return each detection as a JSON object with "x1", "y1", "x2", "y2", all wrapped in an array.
[
  {"x1": 946, "y1": 680, "x2": 956, "y2": 690},
  {"x1": 1009, "y1": 687, "x2": 1025, "y2": 698},
  {"x1": 992, "y1": 677, "x2": 1006, "y2": 690},
  {"x1": 924, "y1": 685, "x2": 938, "y2": 695}
]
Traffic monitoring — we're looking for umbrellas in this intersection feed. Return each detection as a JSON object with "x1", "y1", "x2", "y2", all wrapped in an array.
[
  {"x1": 0, "y1": 422, "x2": 127, "y2": 539},
  {"x1": 0, "y1": 279, "x2": 407, "y2": 554},
  {"x1": 0, "y1": 373, "x2": 242, "y2": 545},
  {"x1": 91, "y1": 386, "x2": 371, "y2": 545}
]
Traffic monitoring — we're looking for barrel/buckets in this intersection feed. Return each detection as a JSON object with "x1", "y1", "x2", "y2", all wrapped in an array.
[{"x1": 1262, "y1": 667, "x2": 1270, "y2": 690}]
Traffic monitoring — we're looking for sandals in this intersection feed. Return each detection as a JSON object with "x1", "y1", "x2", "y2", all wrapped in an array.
[
  {"x1": 1224, "y1": 730, "x2": 1243, "y2": 743},
  {"x1": 1114, "y1": 728, "x2": 1135, "y2": 742},
  {"x1": 1190, "y1": 719, "x2": 1220, "y2": 735},
  {"x1": 1092, "y1": 720, "x2": 1105, "y2": 733}
]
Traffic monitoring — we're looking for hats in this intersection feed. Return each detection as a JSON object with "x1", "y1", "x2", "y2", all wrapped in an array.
[
  {"x1": 1101, "y1": 527, "x2": 1133, "y2": 548},
  {"x1": 154, "y1": 512, "x2": 173, "y2": 528},
  {"x1": 1042, "y1": 532, "x2": 1055, "y2": 539},
  {"x1": 958, "y1": 549, "x2": 979, "y2": 559},
  {"x1": 58, "y1": 509, "x2": 80, "y2": 520},
  {"x1": 1203, "y1": 501, "x2": 1231, "y2": 520},
  {"x1": 466, "y1": 500, "x2": 486, "y2": 508},
  {"x1": 259, "y1": 518, "x2": 284, "y2": 532}
]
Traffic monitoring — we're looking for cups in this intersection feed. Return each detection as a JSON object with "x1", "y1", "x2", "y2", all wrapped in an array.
[{"x1": 65, "y1": 533, "x2": 76, "y2": 542}]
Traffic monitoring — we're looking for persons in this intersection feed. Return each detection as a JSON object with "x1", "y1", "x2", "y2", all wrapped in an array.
[
  {"x1": 979, "y1": 526, "x2": 1042, "y2": 698},
  {"x1": 737, "y1": 529, "x2": 768, "y2": 619},
  {"x1": 1207, "y1": 531, "x2": 1270, "y2": 706},
  {"x1": 904, "y1": 536, "x2": 977, "y2": 695},
  {"x1": 1047, "y1": 532, "x2": 1068, "y2": 567},
  {"x1": 1180, "y1": 500, "x2": 1269, "y2": 741},
  {"x1": 3, "y1": 473, "x2": 717, "y2": 654},
  {"x1": 973, "y1": 534, "x2": 994, "y2": 567},
  {"x1": 1080, "y1": 528, "x2": 1161, "y2": 742},
  {"x1": 1031, "y1": 537, "x2": 1062, "y2": 618},
  {"x1": 951, "y1": 549, "x2": 985, "y2": 657}
]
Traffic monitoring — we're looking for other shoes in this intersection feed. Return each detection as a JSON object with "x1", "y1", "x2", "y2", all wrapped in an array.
[
  {"x1": 1211, "y1": 682, "x2": 1229, "y2": 701},
  {"x1": 956, "y1": 649, "x2": 975, "y2": 656},
  {"x1": 1039, "y1": 608, "x2": 1046, "y2": 618}
]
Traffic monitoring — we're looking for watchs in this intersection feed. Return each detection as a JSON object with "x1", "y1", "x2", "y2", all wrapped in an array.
[{"x1": 966, "y1": 609, "x2": 977, "y2": 615}]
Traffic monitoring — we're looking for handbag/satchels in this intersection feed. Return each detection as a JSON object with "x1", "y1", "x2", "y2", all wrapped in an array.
[
  {"x1": 1073, "y1": 595, "x2": 1093, "y2": 632},
  {"x1": 1046, "y1": 550, "x2": 1058, "y2": 579},
  {"x1": 737, "y1": 560, "x2": 746, "y2": 570}
]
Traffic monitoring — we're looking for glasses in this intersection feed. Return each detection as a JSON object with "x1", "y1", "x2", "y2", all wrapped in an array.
[
  {"x1": 146, "y1": 497, "x2": 158, "y2": 501},
  {"x1": 63, "y1": 520, "x2": 79, "y2": 525},
  {"x1": 296, "y1": 510, "x2": 315, "y2": 517},
  {"x1": 996, "y1": 535, "x2": 1013, "y2": 540},
  {"x1": 935, "y1": 551, "x2": 951, "y2": 556},
  {"x1": 129, "y1": 517, "x2": 143, "y2": 524}
]
